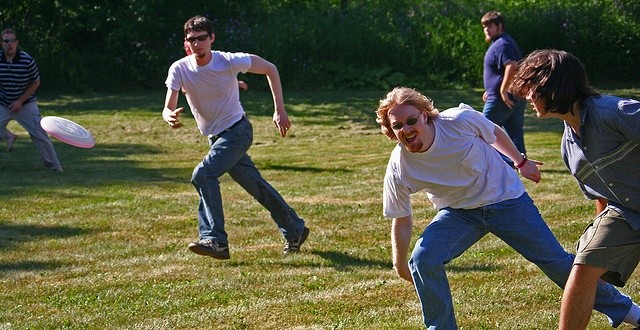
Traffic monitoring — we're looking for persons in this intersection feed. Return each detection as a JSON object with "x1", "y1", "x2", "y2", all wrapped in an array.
[
  {"x1": 374, "y1": 86, "x2": 639, "y2": 329},
  {"x1": 0, "y1": 27, "x2": 66, "y2": 173},
  {"x1": 506, "y1": 48, "x2": 639, "y2": 330},
  {"x1": 183, "y1": 36, "x2": 249, "y2": 92},
  {"x1": 480, "y1": 11, "x2": 527, "y2": 154},
  {"x1": 162, "y1": 16, "x2": 309, "y2": 260}
]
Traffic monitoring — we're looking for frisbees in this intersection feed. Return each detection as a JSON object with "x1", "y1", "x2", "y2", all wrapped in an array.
[{"x1": 40, "y1": 115, "x2": 95, "y2": 149}]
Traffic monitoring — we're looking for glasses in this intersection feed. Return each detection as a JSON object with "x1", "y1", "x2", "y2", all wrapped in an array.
[
  {"x1": 388, "y1": 111, "x2": 424, "y2": 129},
  {"x1": 2, "y1": 37, "x2": 17, "y2": 43},
  {"x1": 185, "y1": 30, "x2": 212, "y2": 43}
]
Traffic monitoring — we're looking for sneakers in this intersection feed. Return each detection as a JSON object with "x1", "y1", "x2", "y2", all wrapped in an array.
[
  {"x1": 6, "y1": 134, "x2": 17, "y2": 152},
  {"x1": 188, "y1": 238, "x2": 230, "y2": 260},
  {"x1": 283, "y1": 226, "x2": 309, "y2": 255}
]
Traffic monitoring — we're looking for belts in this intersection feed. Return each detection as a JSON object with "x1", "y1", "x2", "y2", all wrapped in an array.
[{"x1": 207, "y1": 116, "x2": 247, "y2": 143}]
